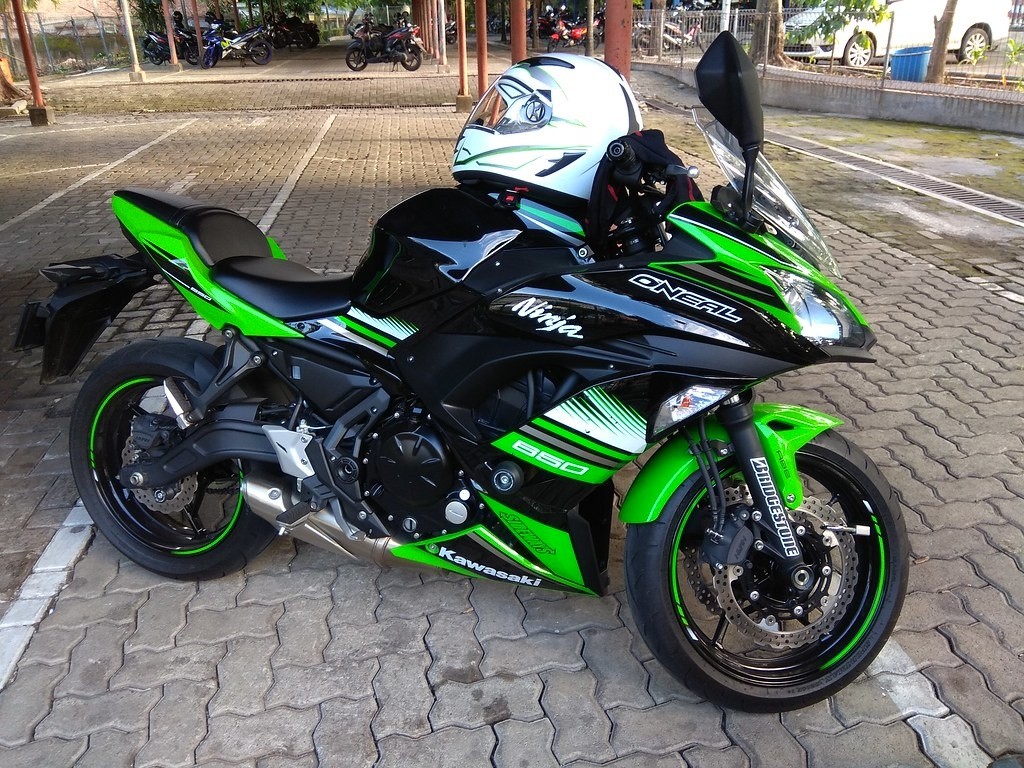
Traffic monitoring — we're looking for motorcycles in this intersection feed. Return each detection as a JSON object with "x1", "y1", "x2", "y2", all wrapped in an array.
[
  {"x1": 200, "y1": 13, "x2": 278, "y2": 69},
  {"x1": 345, "y1": 4, "x2": 457, "y2": 72},
  {"x1": 140, "y1": 11, "x2": 200, "y2": 65},
  {"x1": 487, "y1": 3, "x2": 606, "y2": 54},
  {"x1": 245, "y1": 11, "x2": 320, "y2": 49},
  {"x1": 633, "y1": 8, "x2": 706, "y2": 57},
  {"x1": 9, "y1": 30, "x2": 910, "y2": 715}
]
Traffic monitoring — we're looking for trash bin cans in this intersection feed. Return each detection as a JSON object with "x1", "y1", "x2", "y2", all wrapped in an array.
[{"x1": 889, "y1": 46, "x2": 932, "y2": 83}]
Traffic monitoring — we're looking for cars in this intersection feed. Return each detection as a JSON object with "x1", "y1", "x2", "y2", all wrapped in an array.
[{"x1": 783, "y1": 0, "x2": 1012, "y2": 68}]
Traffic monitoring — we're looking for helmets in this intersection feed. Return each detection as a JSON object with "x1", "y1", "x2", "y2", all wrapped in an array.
[
  {"x1": 546, "y1": 7, "x2": 552, "y2": 11},
  {"x1": 453, "y1": 50, "x2": 641, "y2": 200},
  {"x1": 172, "y1": 11, "x2": 183, "y2": 22}
]
